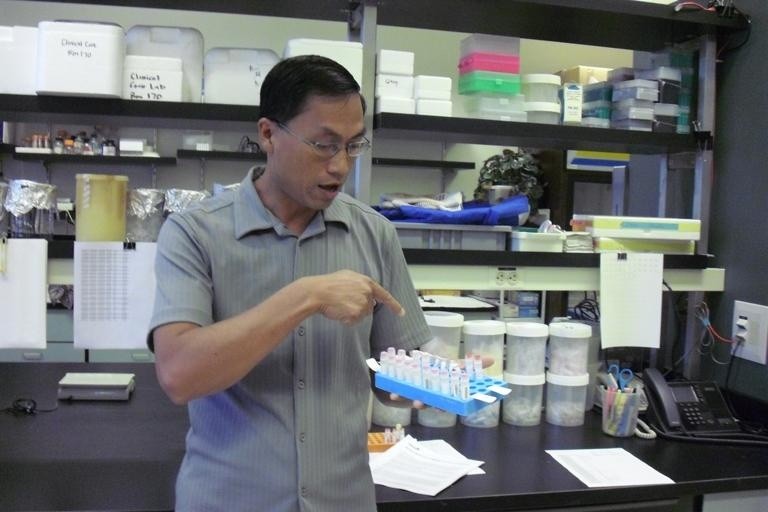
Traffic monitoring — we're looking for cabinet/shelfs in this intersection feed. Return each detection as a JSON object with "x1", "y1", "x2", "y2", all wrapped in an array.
[
  {"x1": 0, "y1": 310, "x2": 86, "y2": 360},
  {"x1": 86, "y1": 347, "x2": 155, "y2": 362},
  {"x1": 367, "y1": 1, "x2": 754, "y2": 380},
  {"x1": 0, "y1": 0, "x2": 367, "y2": 304}
]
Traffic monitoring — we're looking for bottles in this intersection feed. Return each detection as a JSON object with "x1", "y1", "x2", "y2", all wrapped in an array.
[
  {"x1": 368, "y1": 309, "x2": 600, "y2": 427},
  {"x1": 384, "y1": 423, "x2": 405, "y2": 443}
]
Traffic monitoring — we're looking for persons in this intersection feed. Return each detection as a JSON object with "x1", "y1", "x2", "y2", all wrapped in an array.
[{"x1": 145, "y1": 54, "x2": 495, "y2": 512}]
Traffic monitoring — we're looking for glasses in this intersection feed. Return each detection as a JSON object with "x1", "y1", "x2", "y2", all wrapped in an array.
[{"x1": 275, "y1": 122, "x2": 371, "y2": 157}]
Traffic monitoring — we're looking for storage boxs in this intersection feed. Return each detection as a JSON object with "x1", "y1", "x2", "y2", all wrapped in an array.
[
  {"x1": 632, "y1": 47, "x2": 696, "y2": 134},
  {"x1": 0, "y1": 23, "x2": 36, "y2": 98},
  {"x1": 125, "y1": 24, "x2": 206, "y2": 102},
  {"x1": 458, "y1": 52, "x2": 520, "y2": 74},
  {"x1": 415, "y1": 100, "x2": 452, "y2": 117},
  {"x1": 378, "y1": 98, "x2": 415, "y2": 115},
  {"x1": 612, "y1": 79, "x2": 658, "y2": 101},
  {"x1": 519, "y1": 73, "x2": 561, "y2": 102},
  {"x1": 30, "y1": 18, "x2": 123, "y2": 97},
  {"x1": 465, "y1": 109, "x2": 528, "y2": 122},
  {"x1": 462, "y1": 92, "x2": 525, "y2": 113},
  {"x1": 284, "y1": 38, "x2": 362, "y2": 97},
  {"x1": 607, "y1": 67, "x2": 636, "y2": 82},
  {"x1": 205, "y1": 46, "x2": 282, "y2": 112},
  {"x1": 413, "y1": 75, "x2": 452, "y2": 101},
  {"x1": 378, "y1": 49, "x2": 414, "y2": 75},
  {"x1": 612, "y1": 99, "x2": 654, "y2": 120},
  {"x1": 524, "y1": 102, "x2": 560, "y2": 124},
  {"x1": 376, "y1": 76, "x2": 414, "y2": 99},
  {"x1": 459, "y1": 33, "x2": 520, "y2": 58},
  {"x1": 614, "y1": 119, "x2": 652, "y2": 132},
  {"x1": 553, "y1": 65, "x2": 615, "y2": 129},
  {"x1": 459, "y1": 70, "x2": 521, "y2": 96},
  {"x1": 414, "y1": 293, "x2": 496, "y2": 365}
]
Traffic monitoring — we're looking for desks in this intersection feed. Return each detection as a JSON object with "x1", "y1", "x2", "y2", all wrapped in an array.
[
  {"x1": 368, "y1": 372, "x2": 768, "y2": 511},
  {"x1": 0, "y1": 362, "x2": 190, "y2": 510}
]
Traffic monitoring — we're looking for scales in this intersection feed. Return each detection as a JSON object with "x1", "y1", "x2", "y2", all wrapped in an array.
[{"x1": 56, "y1": 372, "x2": 135, "y2": 401}]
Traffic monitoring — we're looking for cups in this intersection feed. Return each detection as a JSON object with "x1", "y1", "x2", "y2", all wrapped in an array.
[{"x1": 602, "y1": 386, "x2": 639, "y2": 438}]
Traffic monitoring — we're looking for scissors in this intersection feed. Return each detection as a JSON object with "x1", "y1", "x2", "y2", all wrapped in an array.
[{"x1": 607, "y1": 364, "x2": 634, "y2": 393}]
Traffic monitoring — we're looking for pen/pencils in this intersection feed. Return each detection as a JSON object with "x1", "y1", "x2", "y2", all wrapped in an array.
[{"x1": 602, "y1": 372, "x2": 637, "y2": 436}]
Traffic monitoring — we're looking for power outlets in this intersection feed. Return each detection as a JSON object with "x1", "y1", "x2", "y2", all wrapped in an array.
[{"x1": 486, "y1": 264, "x2": 524, "y2": 291}]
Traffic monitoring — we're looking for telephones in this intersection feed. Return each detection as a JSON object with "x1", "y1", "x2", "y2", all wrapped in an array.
[{"x1": 642, "y1": 368, "x2": 742, "y2": 435}]
